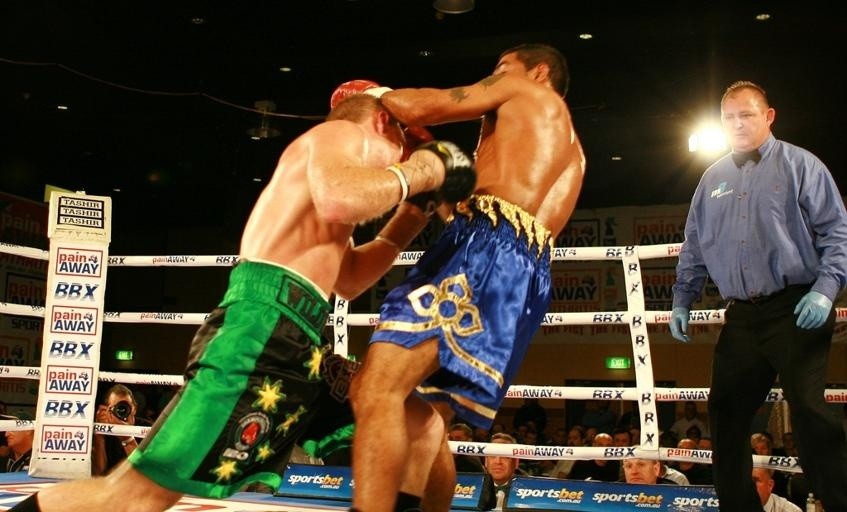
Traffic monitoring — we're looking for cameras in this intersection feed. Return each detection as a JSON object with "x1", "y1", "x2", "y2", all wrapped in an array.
[{"x1": 110, "y1": 400, "x2": 132, "y2": 420}]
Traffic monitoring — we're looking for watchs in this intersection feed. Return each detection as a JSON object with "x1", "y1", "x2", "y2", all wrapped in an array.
[{"x1": 120, "y1": 436, "x2": 135, "y2": 447}]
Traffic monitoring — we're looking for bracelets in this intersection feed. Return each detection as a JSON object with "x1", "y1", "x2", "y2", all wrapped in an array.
[
  {"x1": 384, "y1": 164, "x2": 410, "y2": 204},
  {"x1": 375, "y1": 234, "x2": 401, "y2": 255}
]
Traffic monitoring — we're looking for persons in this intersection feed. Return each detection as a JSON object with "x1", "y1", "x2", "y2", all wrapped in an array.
[
  {"x1": 10, "y1": 93, "x2": 472, "y2": 511},
  {"x1": 93, "y1": 383, "x2": 155, "y2": 477},
  {"x1": 668, "y1": 81, "x2": 846, "y2": 512},
  {"x1": 0, "y1": 401, "x2": 34, "y2": 473},
  {"x1": 328, "y1": 42, "x2": 588, "y2": 511}
]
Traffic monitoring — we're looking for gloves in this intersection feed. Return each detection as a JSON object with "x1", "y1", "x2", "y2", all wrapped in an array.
[
  {"x1": 403, "y1": 125, "x2": 476, "y2": 216},
  {"x1": 330, "y1": 79, "x2": 394, "y2": 110},
  {"x1": 793, "y1": 290, "x2": 833, "y2": 331},
  {"x1": 669, "y1": 307, "x2": 692, "y2": 344}
]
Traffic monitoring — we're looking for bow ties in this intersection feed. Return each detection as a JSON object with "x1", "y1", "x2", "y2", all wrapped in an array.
[
  {"x1": 493, "y1": 484, "x2": 510, "y2": 494},
  {"x1": 731, "y1": 148, "x2": 761, "y2": 168}
]
